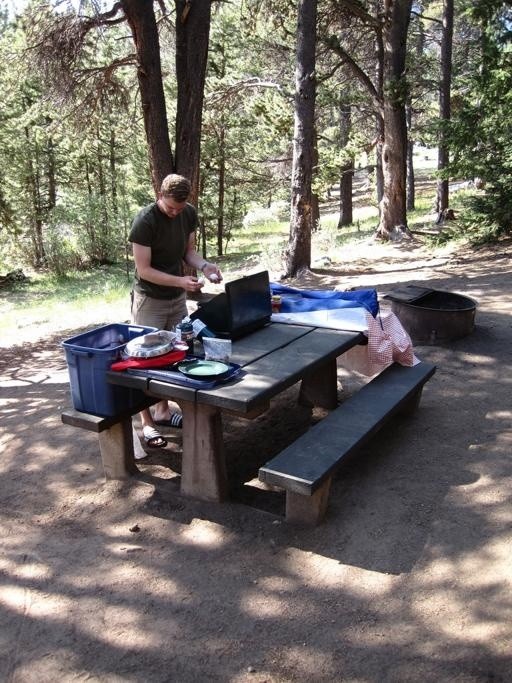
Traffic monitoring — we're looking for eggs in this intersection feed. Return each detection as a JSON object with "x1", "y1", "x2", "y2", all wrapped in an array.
[
  {"x1": 209, "y1": 274, "x2": 219, "y2": 281},
  {"x1": 198, "y1": 278, "x2": 205, "y2": 285}
]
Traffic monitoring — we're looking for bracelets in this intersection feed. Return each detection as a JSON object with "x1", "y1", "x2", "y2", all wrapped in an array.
[{"x1": 199, "y1": 261, "x2": 208, "y2": 272}]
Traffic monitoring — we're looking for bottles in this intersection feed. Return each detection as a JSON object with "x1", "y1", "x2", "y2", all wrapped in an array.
[{"x1": 174, "y1": 315, "x2": 217, "y2": 355}]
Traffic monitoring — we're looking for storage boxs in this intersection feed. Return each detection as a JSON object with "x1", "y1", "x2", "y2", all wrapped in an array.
[{"x1": 62, "y1": 323, "x2": 159, "y2": 417}]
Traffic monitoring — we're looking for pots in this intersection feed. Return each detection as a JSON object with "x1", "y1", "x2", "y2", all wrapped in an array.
[{"x1": 382, "y1": 285, "x2": 478, "y2": 344}]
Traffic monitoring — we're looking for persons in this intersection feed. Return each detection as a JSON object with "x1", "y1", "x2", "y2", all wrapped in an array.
[{"x1": 128, "y1": 175, "x2": 223, "y2": 450}]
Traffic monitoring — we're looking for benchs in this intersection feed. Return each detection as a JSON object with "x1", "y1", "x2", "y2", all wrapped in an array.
[
  {"x1": 258, "y1": 360, "x2": 435, "y2": 525},
  {"x1": 62, "y1": 394, "x2": 162, "y2": 480}
]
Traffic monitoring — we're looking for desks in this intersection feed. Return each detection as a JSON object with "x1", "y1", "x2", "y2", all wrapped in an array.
[{"x1": 103, "y1": 320, "x2": 364, "y2": 504}]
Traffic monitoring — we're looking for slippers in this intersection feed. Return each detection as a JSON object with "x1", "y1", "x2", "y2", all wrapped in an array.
[
  {"x1": 144, "y1": 431, "x2": 166, "y2": 447},
  {"x1": 155, "y1": 413, "x2": 183, "y2": 429}
]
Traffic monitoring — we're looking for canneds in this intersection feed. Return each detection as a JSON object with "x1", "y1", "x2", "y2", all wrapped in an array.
[{"x1": 179, "y1": 323, "x2": 194, "y2": 353}]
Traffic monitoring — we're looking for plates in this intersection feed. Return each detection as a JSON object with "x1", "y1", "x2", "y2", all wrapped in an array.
[
  {"x1": 177, "y1": 358, "x2": 230, "y2": 377},
  {"x1": 123, "y1": 332, "x2": 174, "y2": 358}
]
